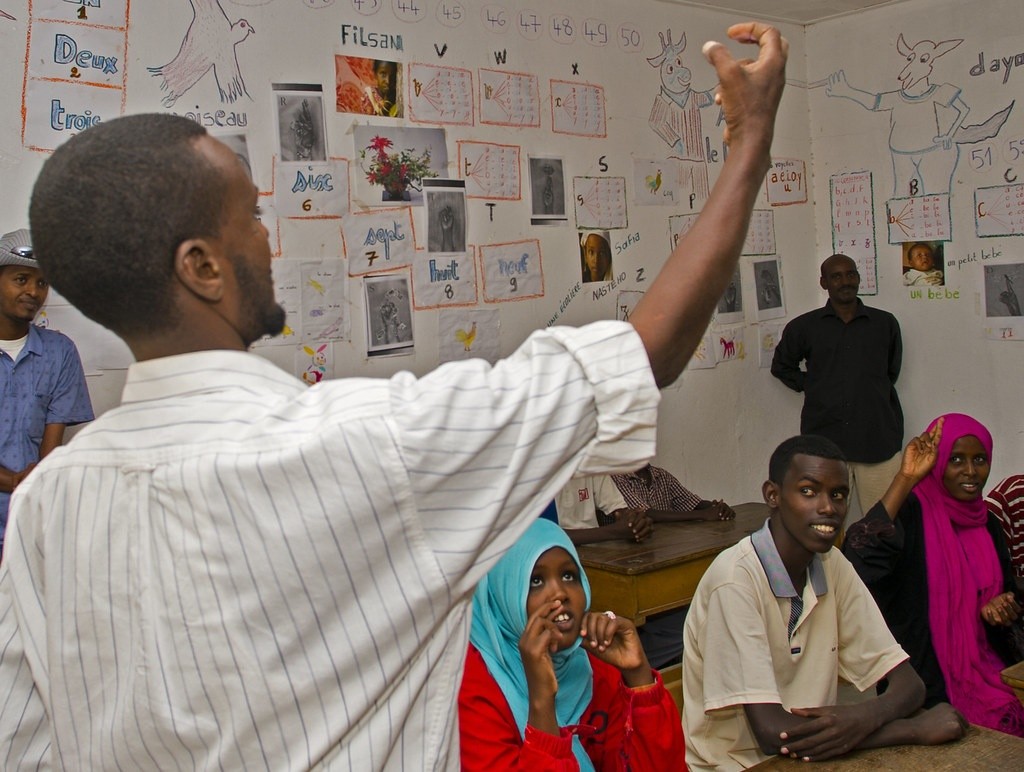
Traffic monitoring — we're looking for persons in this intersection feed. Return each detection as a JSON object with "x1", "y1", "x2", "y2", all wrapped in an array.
[
  {"x1": 841, "y1": 413, "x2": 1024, "y2": 738},
  {"x1": 577, "y1": 232, "x2": 612, "y2": 284},
  {"x1": 904, "y1": 243, "x2": 945, "y2": 285},
  {"x1": 2, "y1": 228, "x2": 95, "y2": 556},
  {"x1": 539, "y1": 465, "x2": 736, "y2": 547},
  {"x1": 0, "y1": 22, "x2": 791, "y2": 772},
  {"x1": 770, "y1": 253, "x2": 908, "y2": 519},
  {"x1": 458, "y1": 517, "x2": 687, "y2": 771},
  {"x1": 682, "y1": 433, "x2": 971, "y2": 771},
  {"x1": 364, "y1": 60, "x2": 399, "y2": 116}
]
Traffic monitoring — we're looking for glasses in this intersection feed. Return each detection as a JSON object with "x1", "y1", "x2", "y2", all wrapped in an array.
[{"x1": 10, "y1": 246, "x2": 36, "y2": 259}]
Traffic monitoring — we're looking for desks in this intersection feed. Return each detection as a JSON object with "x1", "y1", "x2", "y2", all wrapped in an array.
[
  {"x1": 1000, "y1": 658, "x2": 1024, "y2": 708},
  {"x1": 740, "y1": 708, "x2": 1024, "y2": 772},
  {"x1": 574, "y1": 501, "x2": 845, "y2": 630}
]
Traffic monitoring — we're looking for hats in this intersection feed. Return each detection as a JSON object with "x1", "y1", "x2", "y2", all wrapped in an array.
[{"x1": 0, "y1": 228, "x2": 41, "y2": 268}]
